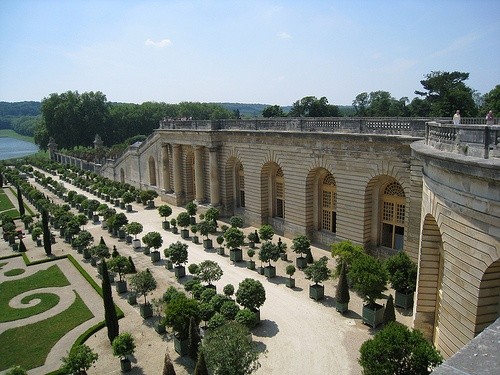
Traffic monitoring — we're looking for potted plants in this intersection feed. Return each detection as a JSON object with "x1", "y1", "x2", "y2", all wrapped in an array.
[
  {"x1": 335, "y1": 260, "x2": 350, "y2": 314},
  {"x1": 109, "y1": 254, "x2": 131, "y2": 293},
  {"x1": 128, "y1": 282, "x2": 137, "y2": 305},
  {"x1": 285, "y1": 265, "x2": 296, "y2": 288},
  {"x1": 235, "y1": 309, "x2": 257, "y2": 344},
  {"x1": 128, "y1": 269, "x2": 158, "y2": 319},
  {"x1": 384, "y1": 249, "x2": 417, "y2": 311},
  {"x1": 348, "y1": 253, "x2": 387, "y2": 329},
  {"x1": 234, "y1": 277, "x2": 265, "y2": 325},
  {"x1": 328, "y1": 240, "x2": 362, "y2": 288},
  {"x1": 0, "y1": 158, "x2": 288, "y2": 282},
  {"x1": 196, "y1": 260, "x2": 223, "y2": 294},
  {"x1": 164, "y1": 291, "x2": 200, "y2": 357},
  {"x1": 302, "y1": 255, "x2": 332, "y2": 302},
  {"x1": 199, "y1": 303, "x2": 215, "y2": 337},
  {"x1": 151, "y1": 296, "x2": 167, "y2": 334},
  {"x1": 111, "y1": 332, "x2": 137, "y2": 373},
  {"x1": 59, "y1": 343, "x2": 98, "y2": 375},
  {"x1": 289, "y1": 234, "x2": 312, "y2": 270},
  {"x1": 187, "y1": 315, "x2": 202, "y2": 369}
]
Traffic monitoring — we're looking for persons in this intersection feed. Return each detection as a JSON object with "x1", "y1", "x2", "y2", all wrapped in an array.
[
  {"x1": 486, "y1": 110, "x2": 494, "y2": 125},
  {"x1": 453, "y1": 109, "x2": 461, "y2": 125}
]
